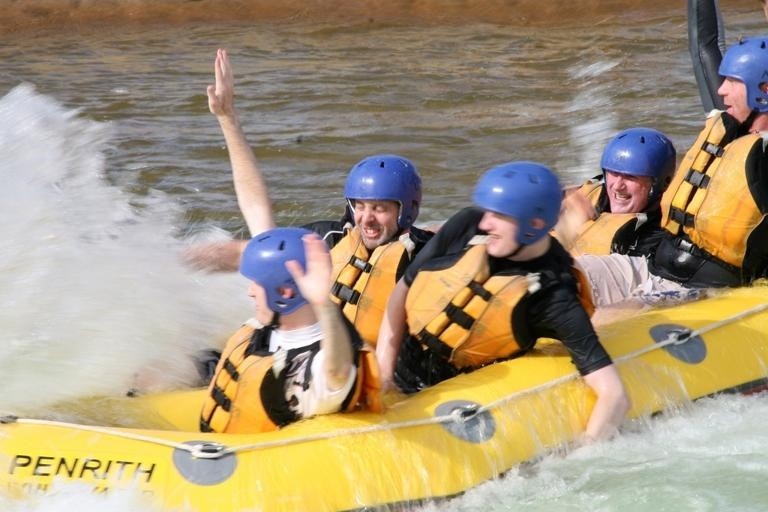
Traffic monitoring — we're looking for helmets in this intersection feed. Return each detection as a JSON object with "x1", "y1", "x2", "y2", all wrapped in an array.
[
  {"x1": 599, "y1": 126, "x2": 677, "y2": 199},
  {"x1": 718, "y1": 33, "x2": 768, "y2": 112},
  {"x1": 473, "y1": 160, "x2": 562, "y2": 246},
  {"x1": 240, "y1": 226, "x2": 331, "y2": 315},
  {"x1": 343, "y1": 155, "x2": 421, "y2": 230}
]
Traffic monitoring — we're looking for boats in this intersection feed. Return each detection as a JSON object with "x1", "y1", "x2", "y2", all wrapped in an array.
[{"x1": 0, "y1": 285, "x2": 768, "y2": 512}]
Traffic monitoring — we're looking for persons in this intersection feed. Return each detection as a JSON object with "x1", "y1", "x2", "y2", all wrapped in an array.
[
  {"x1": 574, "y1": 32, "x2": 768, "y2": 332},
  {"x1": 179, "y1": 154, "x2": 597, "y2": 370},
  {"x1": 541, "y1": 126, "x2": 676, "y2": 257},
  {"x1": 95, "y1": 42, "x2": 380, "y2": 434},
  {"x1": 373, "y1": 160, "x2": 634, "y2": 451}
]
